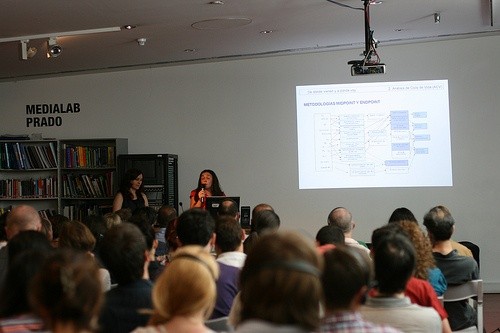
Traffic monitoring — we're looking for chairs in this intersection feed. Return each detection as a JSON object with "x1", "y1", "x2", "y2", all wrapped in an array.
[{"x1": 444, "y1": 279, "x2": 483, "y2": 333}]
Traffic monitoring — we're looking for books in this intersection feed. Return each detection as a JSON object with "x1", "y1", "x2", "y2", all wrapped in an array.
[
  {"x1": 0, "y1": 206, "x2": 12, "y2": 215},
  {"x1": 64, "y1": 174, "x2": 113, "y2": 198},
  {"x1": 60, "y1": 143, "x2": 115, "y2": 168},
  {"x1": 39, "y1": 209, "x2": 58, "y2": 219},
  {"x1": 0, "y1": 176, "x2": 57, "y2": 198},
  {"x1": 64, "y1": 205, "x2": 113, "y2": 225},
  {"x1": 0, "y1": 142, "x2": 57, "y2": 170}
]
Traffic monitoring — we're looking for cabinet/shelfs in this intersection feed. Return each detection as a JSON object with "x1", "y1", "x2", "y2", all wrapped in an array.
[
  {"x1": 0, "y1": 138, "x2": 57, "y2": 220},
  {"x1": 58, "y1": 137, "x2": 128, "y2": 224}
]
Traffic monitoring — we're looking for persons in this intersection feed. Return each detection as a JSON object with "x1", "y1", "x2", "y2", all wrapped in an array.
[
  {"x1": 362, "y1": 233, "x2": 445, "y2": 333},
  {"x1": 4, "y1": 205, "x2": 42, "y2": 241},
  {"x1": 190, "y1": 170, "x2": 240, "y2": 221},
  {"x1": 112, "y1": 170, "x2": 149, "y2": 212},
  {"x1": 0, "y1": 208, "x2": 168, "y2": 333},
  {"x1": 177, "y1": 207, "x2": 242, "y2": 321},
  {"x1": 236, "y1": 230, "x2": 323, "y2": 333},
  {"x1": 328, "y1": 206, "x2": 374, "y2": 280},
  {"x1": 130, "y1": 244, "x2": 222, "y2": 333},
  {"x1": 317, "y1": 243, "x2": 411, "y2": 333},
  {"x1": 154, "y1": 203, "x2": 487, "y2": 333}
]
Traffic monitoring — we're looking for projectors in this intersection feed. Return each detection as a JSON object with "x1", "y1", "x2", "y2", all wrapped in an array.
[{"x1": 350, "y1": 63, "x2": 386, "y2": 76}]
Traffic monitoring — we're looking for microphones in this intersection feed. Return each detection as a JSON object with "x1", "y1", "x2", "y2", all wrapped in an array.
[{"x1": 201, "y1": 185, "x2": 205, "y2": 203}]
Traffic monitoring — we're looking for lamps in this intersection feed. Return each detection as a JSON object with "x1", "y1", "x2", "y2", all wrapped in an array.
[
  {"x1": 18, "y1": 41, "x2": 37, "y2": 61},
  {"x1": 46, "y1": 38, "x2": 62, "y2": 59}
]
